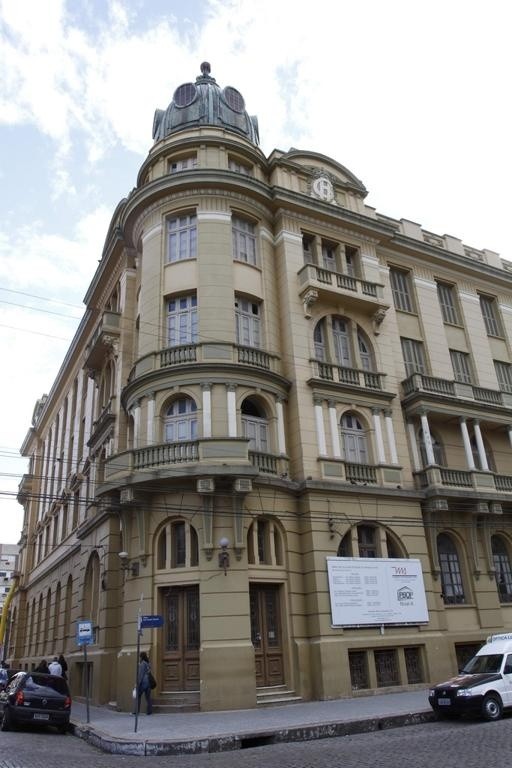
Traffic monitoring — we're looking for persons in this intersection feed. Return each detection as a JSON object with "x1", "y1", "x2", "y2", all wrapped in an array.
[
  {"x1": 127, "y1": 651, "x2": 154, "y2": 718},
  {"x1": 1, "y1": 653, "x2": 68, "y2": 688}
]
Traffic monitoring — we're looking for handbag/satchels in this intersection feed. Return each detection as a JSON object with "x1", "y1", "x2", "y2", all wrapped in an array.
[{"x1": 149, "y1": 673, "x2": 156, "y2": 689}]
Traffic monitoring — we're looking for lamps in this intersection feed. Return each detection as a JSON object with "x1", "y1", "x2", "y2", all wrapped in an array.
[
  {"x1": 216, "y1": 537, "x2": 231, "y2": 576},
  {"x1": 117, "y1": 551, "x2": 140, "y2": 577}
]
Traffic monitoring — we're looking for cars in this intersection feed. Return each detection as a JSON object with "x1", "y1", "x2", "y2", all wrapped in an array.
[
  {"x1": 0, "y1": 671, "x2": 72, "y2": 734},
  {"x1": 0, "y1": 668, "x2": 25, "y2": 694}
]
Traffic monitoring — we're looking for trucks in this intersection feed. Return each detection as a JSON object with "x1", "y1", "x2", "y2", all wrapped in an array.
[{"x1": 428, "y1": 631, "x2": 512, "y2": 722}]
